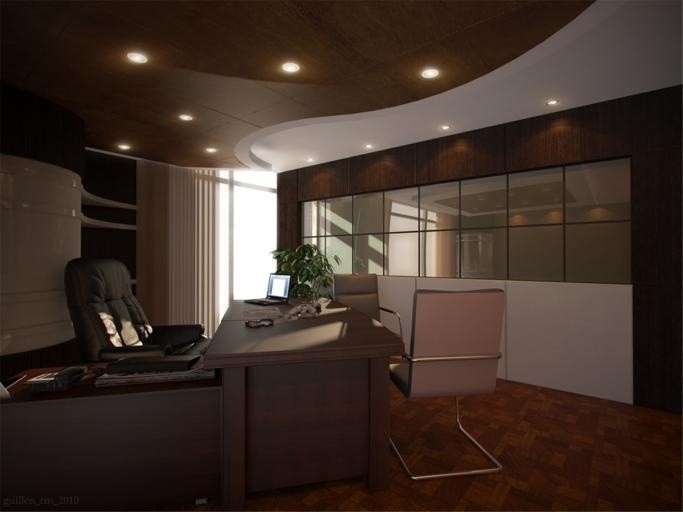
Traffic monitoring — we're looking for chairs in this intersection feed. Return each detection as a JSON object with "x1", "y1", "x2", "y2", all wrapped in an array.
[
  {"x1": 331, "y1": 273, "x2": 506, "y2": 480},
  {"x1": 62, "y1": 255, "x2": 210, "y2": 374}
]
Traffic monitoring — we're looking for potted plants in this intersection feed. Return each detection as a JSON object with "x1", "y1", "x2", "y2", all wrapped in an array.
[{"x1": 272, "y1": 242, "x2": 342, "y2": 319}]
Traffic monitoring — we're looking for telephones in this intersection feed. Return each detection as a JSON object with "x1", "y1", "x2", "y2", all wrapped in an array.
[{"x1": 23, "y1": 366, "x2": 84, "y2": 394}]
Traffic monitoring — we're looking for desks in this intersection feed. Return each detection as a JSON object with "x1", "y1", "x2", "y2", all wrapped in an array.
[
  {"x1": 0, "y1": 365, "x2": 222, "y2": 511},
  {"x1": 204, "y1": 296, "x2": 404, "y2": 510}
]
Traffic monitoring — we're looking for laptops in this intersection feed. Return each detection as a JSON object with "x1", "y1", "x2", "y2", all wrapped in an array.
[{"x1": 244, "y1": 273, "x2": 292, "y2": 306}]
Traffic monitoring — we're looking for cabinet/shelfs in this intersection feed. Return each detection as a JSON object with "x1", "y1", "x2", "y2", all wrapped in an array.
[{"x1": 81, "y1": 186, "x2": 137, "y2": 230}]
